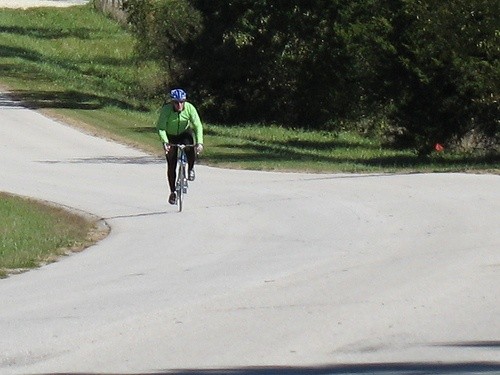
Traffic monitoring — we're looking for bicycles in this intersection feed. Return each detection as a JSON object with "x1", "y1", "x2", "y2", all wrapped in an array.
[{"x1": 165, "y1": 144, "x2": 199, "y2": 212}]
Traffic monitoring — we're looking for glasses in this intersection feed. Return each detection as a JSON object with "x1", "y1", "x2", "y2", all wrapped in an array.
[{"x1": 174, "y1": 101, "x2": 183, "y2": 104}]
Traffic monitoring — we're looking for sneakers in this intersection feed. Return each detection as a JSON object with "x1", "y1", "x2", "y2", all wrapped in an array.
[
  {"x1": 169, "y1": 193, "x2": 176, "y2": 204},
  {"x1": 188, "y1": 169, "x2": 195, "y2": 181}
]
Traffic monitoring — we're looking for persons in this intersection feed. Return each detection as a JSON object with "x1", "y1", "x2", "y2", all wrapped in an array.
[{"x1": 156, "y1": 88, "x2": 203, "y2": 205}]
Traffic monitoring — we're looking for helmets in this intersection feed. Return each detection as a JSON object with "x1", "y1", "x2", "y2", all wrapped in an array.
[{"x1": 171, "y1": 89, "x2": 186, "y2": 101}]
